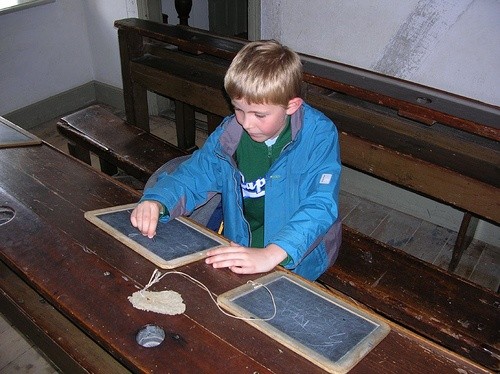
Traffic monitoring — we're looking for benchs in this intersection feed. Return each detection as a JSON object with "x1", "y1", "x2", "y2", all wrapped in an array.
[{"x1": 55, "y1": 18, "x2": 500, "y2": 371}]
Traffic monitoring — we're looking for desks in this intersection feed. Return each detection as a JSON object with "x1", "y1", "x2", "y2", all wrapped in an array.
[{"x1": 0, "y1": 116, "x2": 494, "y2": 374}]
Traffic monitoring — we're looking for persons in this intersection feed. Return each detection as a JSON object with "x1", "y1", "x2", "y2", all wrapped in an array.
[{"x1": 130, "y1": 39, "x2": 343, "y2": 283}]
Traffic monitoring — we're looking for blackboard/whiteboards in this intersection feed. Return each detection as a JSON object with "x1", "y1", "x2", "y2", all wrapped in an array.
[
  {"x1": 83, "y1": 201, "x2": 232, "y2": 269},
  {"x1": 218, "y1": 271, "x2": 392, "y2": 373},
  {"x1": 247, "y1": 0, "x2": 500, "y2": 142}
]
